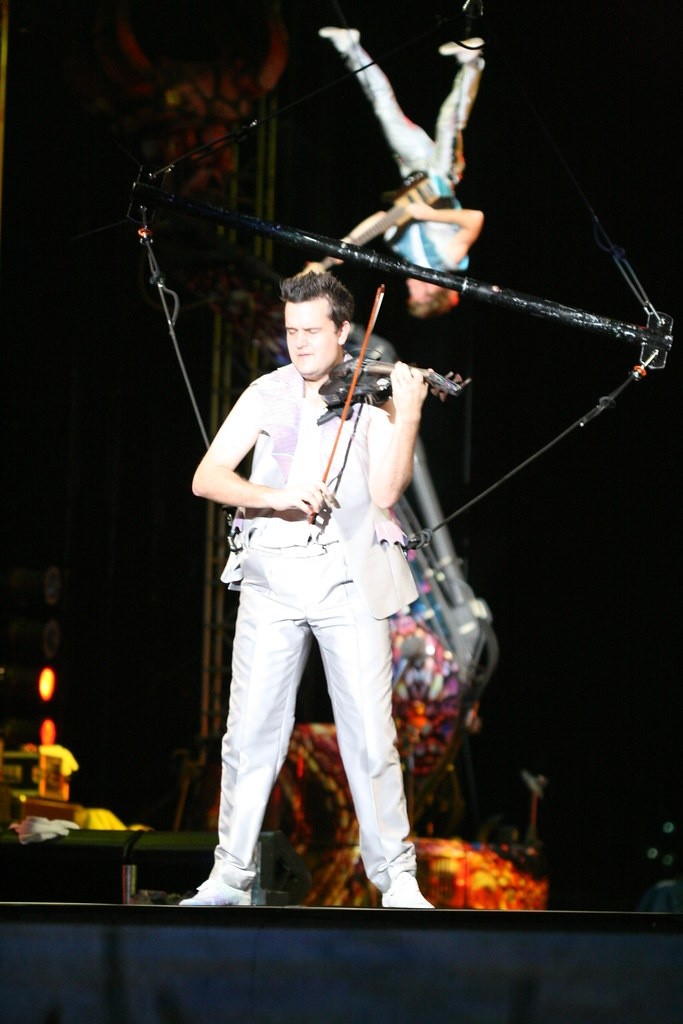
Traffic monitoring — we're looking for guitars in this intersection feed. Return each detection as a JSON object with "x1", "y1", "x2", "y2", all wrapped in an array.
[{"x1": 320, "y1": 167, "x2": 457, "y2": 271}]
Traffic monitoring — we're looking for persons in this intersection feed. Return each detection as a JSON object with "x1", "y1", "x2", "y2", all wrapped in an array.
[
  {"x1": 178, "y1": 270, "x2": 435, "y2": 909},
  {"x1": 318, "y1": 27, "x2": 484, "y2": 319}
]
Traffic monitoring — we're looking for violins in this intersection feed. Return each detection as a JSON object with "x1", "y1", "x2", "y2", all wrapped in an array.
[{"x1": 314, "y1": 354, "x2": 474, "y2": 426}]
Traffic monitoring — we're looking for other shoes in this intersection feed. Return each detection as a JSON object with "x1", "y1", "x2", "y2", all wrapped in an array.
[
  {"x1": 439, "y1": 38, "x2": 484, "y2": 65},
  {"x1": 319, "y1": 28, "x2": 361, "y2": 52},
  {"x1": 382, "y1": 872, "x2": 436, "y2": 909},
  {"x1": 178, "y1": 874, "x2": 253, "y2": 907}
]
